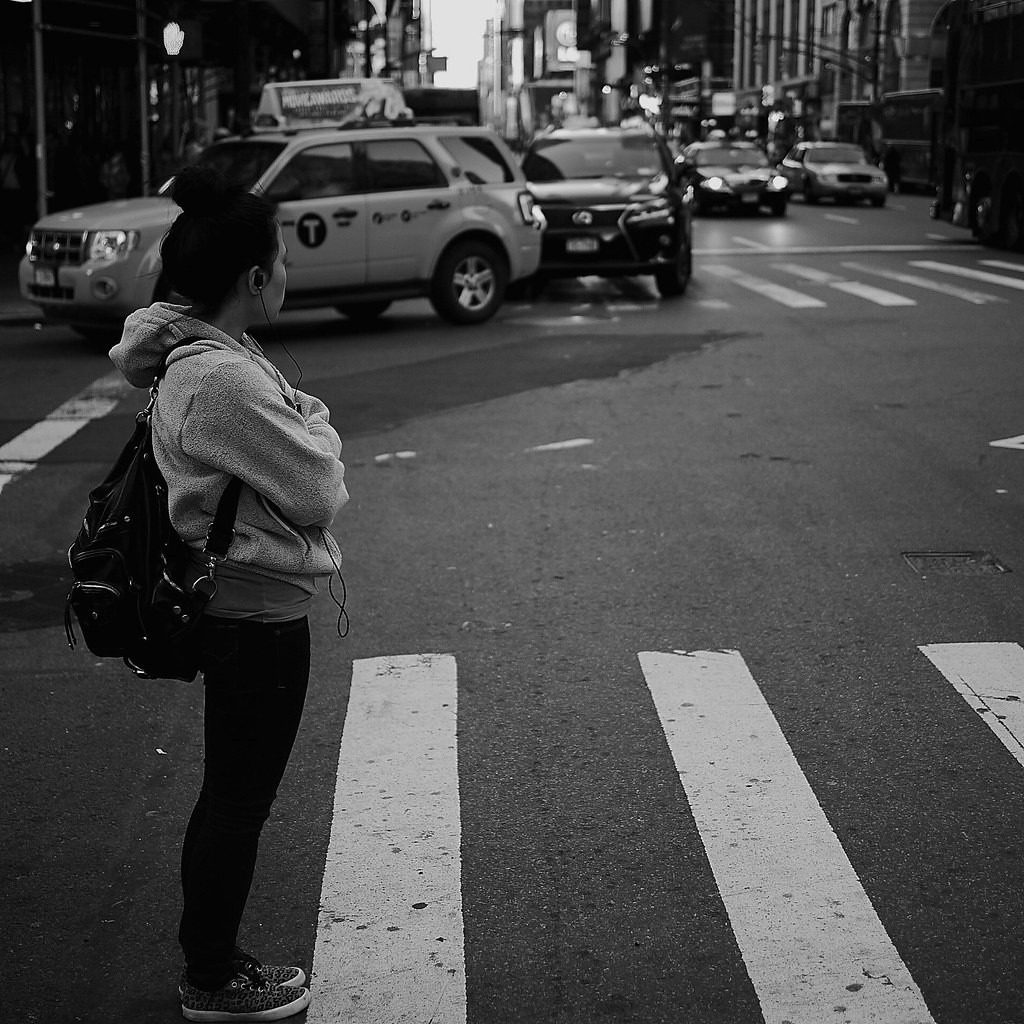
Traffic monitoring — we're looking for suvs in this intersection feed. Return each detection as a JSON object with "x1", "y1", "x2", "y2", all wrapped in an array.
[
  {"x1": 522, "y1": 122, "x2": 698, "y2": 300},
  {"x1": 16, "y1": 123, "x2": 548, "y2": 350}
]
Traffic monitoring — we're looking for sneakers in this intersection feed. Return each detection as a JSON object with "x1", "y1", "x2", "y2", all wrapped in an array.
[
  {"x1": 182, "y1": 967, "x2": 311, "y2": 1022},
  {"x1": 179, "y1": 947, "x2": 306, "y2": 998}
]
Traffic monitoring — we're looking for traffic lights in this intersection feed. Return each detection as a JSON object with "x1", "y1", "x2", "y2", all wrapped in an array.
[{"x1": 160, "y1": 16, "x2": 208, "y2": 68}]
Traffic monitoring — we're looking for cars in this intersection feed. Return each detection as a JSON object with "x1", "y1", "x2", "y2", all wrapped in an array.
[
  {"x1": 674, "y1": 140, "x2": 790, "y2": 219},
  {"x1": 775, "y1": 140, "x2": 890, "y2": 209}
]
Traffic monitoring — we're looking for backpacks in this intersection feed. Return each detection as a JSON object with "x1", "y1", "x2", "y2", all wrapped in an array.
[{"x1": 62, "y1": 341, "x2": 245, "y2": 684}]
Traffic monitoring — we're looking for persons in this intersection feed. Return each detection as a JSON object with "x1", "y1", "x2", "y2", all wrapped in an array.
[{"x1": 109, "y1": 158, "x2": 348, "y2": 1021}]
